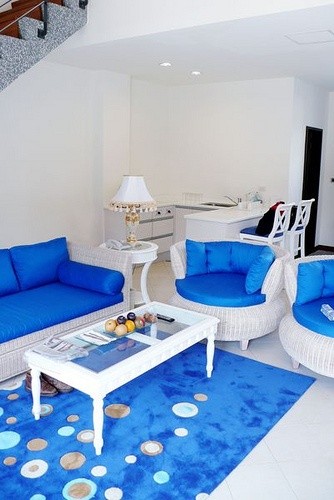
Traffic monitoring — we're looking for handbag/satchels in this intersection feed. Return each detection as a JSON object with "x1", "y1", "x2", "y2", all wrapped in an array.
[{"x1": 256, "y1": 202, "x2": 286, "y2": 237}]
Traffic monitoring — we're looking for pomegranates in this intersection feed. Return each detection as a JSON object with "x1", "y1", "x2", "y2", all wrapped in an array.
[
  {"x1": 135, "y1": 316, "x2": 144, "y2": 328},
  {"x1": 105, "y1": 320, "x2": 127, "y2": 336}
]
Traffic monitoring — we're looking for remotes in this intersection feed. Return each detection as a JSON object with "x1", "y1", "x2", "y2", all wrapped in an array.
[{"x1": 157, "y1": 313, "x2": 175, "y2": 321}]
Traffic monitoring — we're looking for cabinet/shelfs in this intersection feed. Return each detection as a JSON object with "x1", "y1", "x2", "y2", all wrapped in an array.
[{"x1": 137, "y1": 205, "x2": 174, "y2": 255}]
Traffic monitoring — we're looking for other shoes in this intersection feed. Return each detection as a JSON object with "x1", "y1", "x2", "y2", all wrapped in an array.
[
  {"x1": 25, "y1": 369, "x2": 58, "y2": 396},
  {"x1": 41, "y1": 373, "x2": 74, "y2": 393}
]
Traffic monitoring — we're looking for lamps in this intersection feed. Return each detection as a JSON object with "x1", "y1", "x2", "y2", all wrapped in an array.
[{"x1": 109, "y1": 175, "x2": 159, "y2": 248}]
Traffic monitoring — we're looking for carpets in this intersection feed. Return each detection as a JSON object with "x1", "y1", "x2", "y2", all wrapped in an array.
[{"x1": 0, "y1": 327, "x2": 317, "y2": 500}]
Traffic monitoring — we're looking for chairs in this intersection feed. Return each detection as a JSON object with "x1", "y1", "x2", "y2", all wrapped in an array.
[
  {"x1": 170, "y1": 236, "x2": 291, "y2": 351},
  {"x1": 278, "y1": 254, "x2": 334, "y2": 379},
  {"x1": 240, "y1": 199, "x2": 315, "y2": 257}
]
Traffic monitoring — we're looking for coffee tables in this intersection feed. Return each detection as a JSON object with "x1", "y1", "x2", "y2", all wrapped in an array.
[{"x1": 23, "y1": 300, "x2": 221, "y2": 455}]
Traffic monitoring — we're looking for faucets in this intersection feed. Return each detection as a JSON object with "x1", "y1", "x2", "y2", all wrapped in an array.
[{"x1": 224, "y1": 195, "x2": 241, "y2": 205}]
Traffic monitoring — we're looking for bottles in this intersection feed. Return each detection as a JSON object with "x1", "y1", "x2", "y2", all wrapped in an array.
[
  {"x1": 320, "y1": 303, "x2": 334, "y2": 321},
  {"x1": 247, "y1": 192, "x2": 261, "y2": 200}
]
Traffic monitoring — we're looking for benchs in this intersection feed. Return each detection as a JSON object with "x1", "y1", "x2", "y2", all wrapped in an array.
[{"x1": 0, "y1": 237, "x2": 132, "y2": 382}]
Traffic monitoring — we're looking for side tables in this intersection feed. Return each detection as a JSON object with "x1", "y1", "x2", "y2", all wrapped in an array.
[{"x1": 99, "y1": 240, "x2": 159, "y2": 305}]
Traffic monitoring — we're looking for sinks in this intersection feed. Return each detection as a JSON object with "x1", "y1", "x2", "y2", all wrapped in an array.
[{"x1": 198, "y1": 201, "x2": 236, "y2": 207}]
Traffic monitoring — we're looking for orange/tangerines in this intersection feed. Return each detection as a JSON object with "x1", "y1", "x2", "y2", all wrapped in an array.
[{"x1": 125, "y1": 320, "x2": 134, "y2": 331}]
